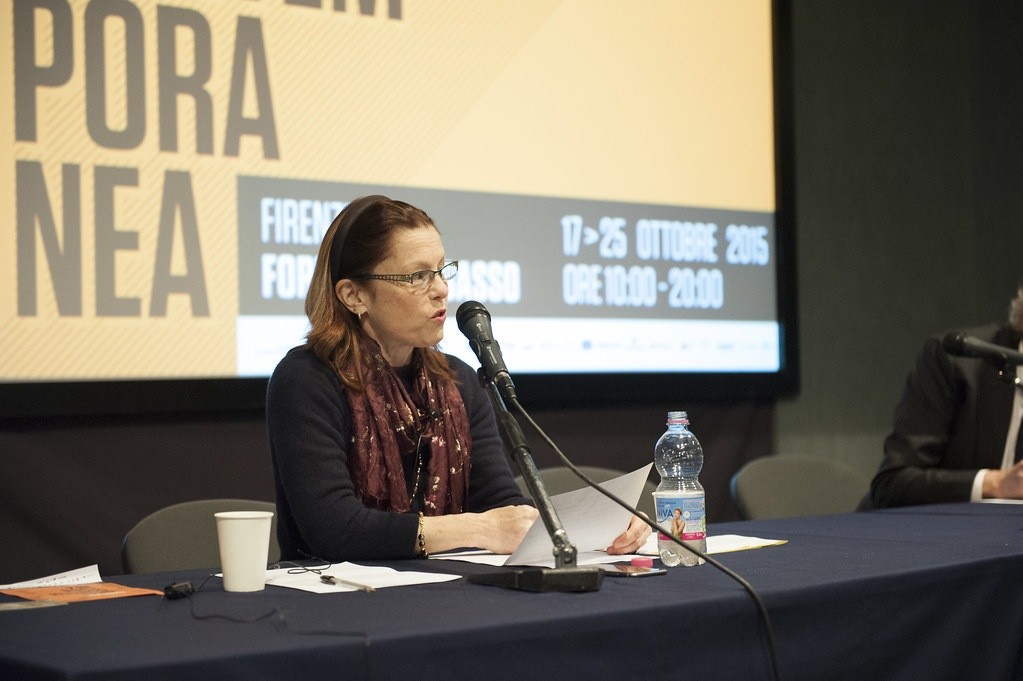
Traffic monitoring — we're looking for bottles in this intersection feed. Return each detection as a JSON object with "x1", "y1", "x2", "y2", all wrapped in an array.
[{"x1": 651, "y1": 410, "x2": 706, "y2": 568}]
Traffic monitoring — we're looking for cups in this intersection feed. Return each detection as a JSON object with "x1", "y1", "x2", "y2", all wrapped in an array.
[{"x1": 214, "y1": 510, "x2": 275, "y2": 593}]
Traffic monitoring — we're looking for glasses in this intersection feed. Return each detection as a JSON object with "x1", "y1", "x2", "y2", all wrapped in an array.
[{"x1": 349, "y1": 258, "x2": 458, "y2": 291}]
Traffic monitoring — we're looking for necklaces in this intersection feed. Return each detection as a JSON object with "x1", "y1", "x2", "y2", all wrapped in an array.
[{"x1": 409, "y1": 453, "x2": 422, "y2": 502}]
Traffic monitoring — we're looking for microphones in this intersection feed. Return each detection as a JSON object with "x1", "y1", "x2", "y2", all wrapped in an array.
[
  {"x1": 456, "y1": 301, "x2": 517, "y2": 400},
  {"x1": 942, "y1": 331, "x2": 1023, "y2": 366}
]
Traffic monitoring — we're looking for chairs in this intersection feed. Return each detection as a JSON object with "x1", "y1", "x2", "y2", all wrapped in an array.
[
  {"x1": 730, "y1": 454, "x2": 870, "y2": 520},
  {"x1": 121, "y1": 500, "x2": 282, "y2": 574},
  {"x1": 512, "y1": 466, "x2": 657, "y2": 523}
]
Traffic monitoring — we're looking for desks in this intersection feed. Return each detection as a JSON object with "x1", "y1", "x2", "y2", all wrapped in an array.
[{"x1": 0, "y1": 500, "x2": 1023, "y2": 681}]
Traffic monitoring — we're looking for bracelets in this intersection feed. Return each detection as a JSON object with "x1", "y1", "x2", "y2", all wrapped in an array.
[{"x1": 418, "y1": 511, "x2": 428, "y2": 560}]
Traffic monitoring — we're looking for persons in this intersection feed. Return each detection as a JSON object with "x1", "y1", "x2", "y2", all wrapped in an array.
[
  {"x1": 266, "y1": 194, "x2": 652, "y2": 565},
  {"x1": 869, "y1": 286, "x2": 1023, "y2": 508},
  {"x1": 671, "y1": 509, "x2": 685, "y2": 540}
]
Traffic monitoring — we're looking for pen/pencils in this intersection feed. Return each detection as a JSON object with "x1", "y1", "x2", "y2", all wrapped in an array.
[{"x1": 320, "y1": 575, "x2": 377, "y2": 593}]
